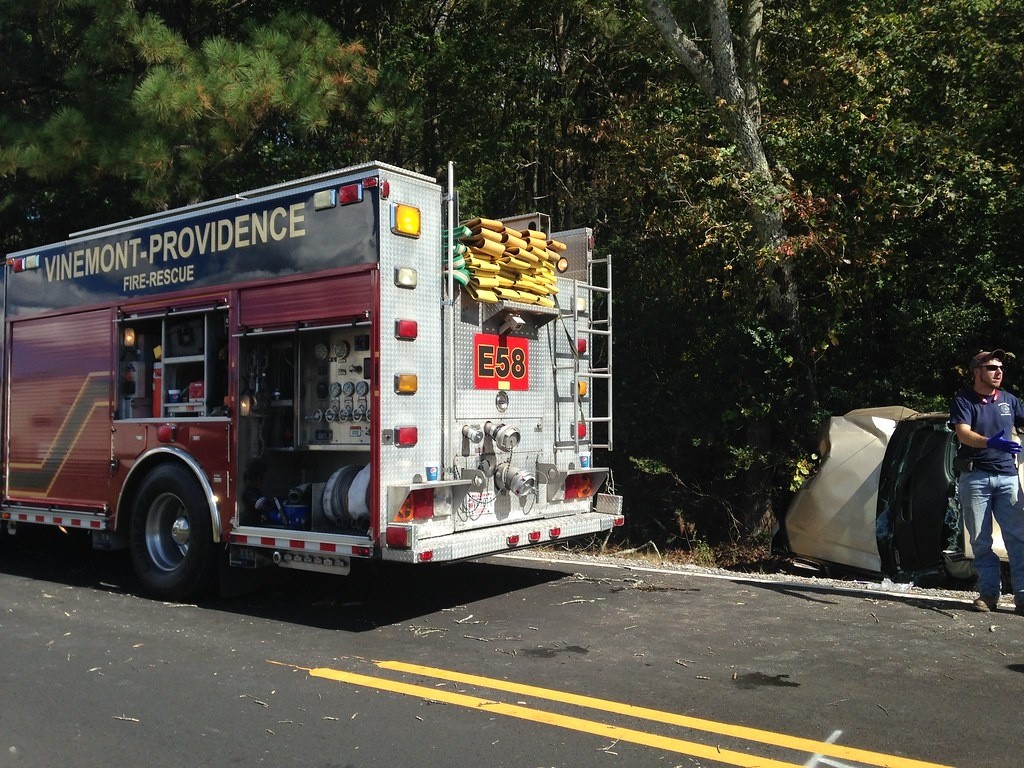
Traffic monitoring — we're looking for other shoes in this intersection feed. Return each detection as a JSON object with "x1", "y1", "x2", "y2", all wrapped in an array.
[
  {"x1": 1014, "y1": 603, "x2": 1024, "y2": 615},
  {"x1": 972, "y1": 595, "x2": 999, "y2": 612}
]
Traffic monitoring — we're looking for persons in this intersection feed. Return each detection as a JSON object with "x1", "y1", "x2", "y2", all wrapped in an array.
[{"x1": 949, "y1": 347, "x2": 1024, "y2": 617}]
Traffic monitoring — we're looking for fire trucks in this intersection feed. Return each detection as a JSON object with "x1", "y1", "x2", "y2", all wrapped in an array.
[{"x1": 0, "y1": 157, "x2": 627, "y2": 605}]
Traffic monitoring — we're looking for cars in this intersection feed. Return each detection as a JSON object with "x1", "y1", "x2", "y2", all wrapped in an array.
[{"x1": 782, "y1": 404, "x2": 1024, "y2": 594}]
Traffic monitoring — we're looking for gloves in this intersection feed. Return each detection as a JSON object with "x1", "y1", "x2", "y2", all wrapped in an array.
[{"x1": 986, "y1": 428, "x2": 1023, "y2": 456}]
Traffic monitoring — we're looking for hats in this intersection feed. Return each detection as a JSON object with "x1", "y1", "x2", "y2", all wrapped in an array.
[{"x1": 968, "y1": 349, "x2": 1007, "y2": 374}]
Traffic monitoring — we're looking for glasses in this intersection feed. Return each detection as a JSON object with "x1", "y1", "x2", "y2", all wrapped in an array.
[{"x1": 978, "y1": 365, "x2": 1006, "y2": 371}]
